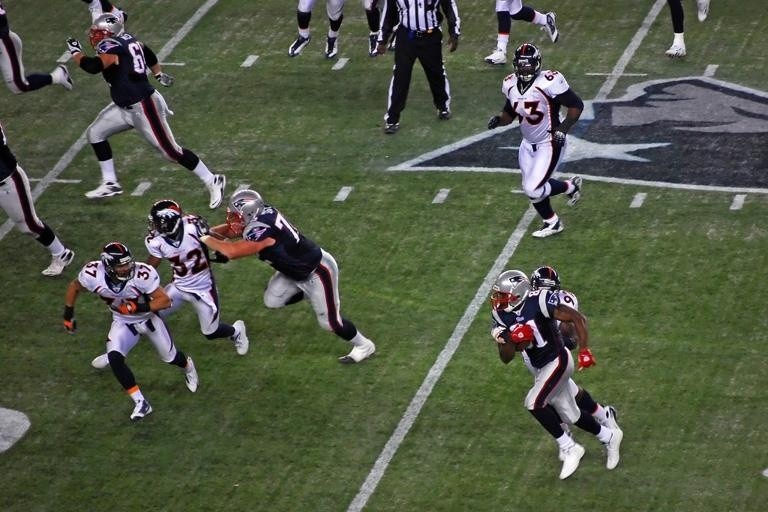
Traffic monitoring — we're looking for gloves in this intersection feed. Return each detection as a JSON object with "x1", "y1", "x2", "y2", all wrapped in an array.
[
  {"x1": 60, "y1": 315, "x2": 78, "y2": 336},
  {"x1": 378, "y1": 43, "x2": 386, "y2": 54},
  {"x1": 546, "y1": 127, "x2": 570, "y2": 145},
  {"x1": 510, "y1": 323, "x2": 534, "y2": 345},
  {"x1": 447, "y1": 35, "x2": 460, "y2": 51},
  {"x1": 486, "y1": 114, "x2": 502, "y2": 132},
  {"x1": 118, "y1": 300, "x2": 140, "y2": 315},
  {"x1": 155, "y1": 71, "x2": 177, "y2": 87},
  {"x1": 65, "y1": 35, "x2": 83, "y2": 56},
  {"x1": 576, "y1": 349, "x2": 597, "y2": 372}
]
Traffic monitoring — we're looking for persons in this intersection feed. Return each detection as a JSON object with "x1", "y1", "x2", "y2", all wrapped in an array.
[
  {"x1": 185, "y1": 189, "x2": 374, "y2": 363},
  {"x1": 530, "y1": 267, "x2": 616, "y2": 461},
  {"x1": 140, "y1": 201, "x2": 249, "y2": 356},
  {"x1": 483, "y1": 43, "x2": 584, "y2": 238},
  {"x1": 489, "y1": 268, "x2": 623, "y2": 479},
  {"x1": 665, "y1": 0, "x2": 711, "y2": 57},
  {"x1": 288, "y1": 0, "x2": 559, "y2": 136},
  {"x1": 0, "y1": 2, "x2": 75, "y2": 93},
  {"x1": 58, "y1": 243, "x2": 199, "y2": 422},
  {"x1": 81, "y1": 0, "x2": 127, "y2": 25},
  {"x1": 0, "y1": 121, "x2": 74, "y2": 277},
  {"x1": 66, "y1": 15, "x2": 225, "y2": 210}
]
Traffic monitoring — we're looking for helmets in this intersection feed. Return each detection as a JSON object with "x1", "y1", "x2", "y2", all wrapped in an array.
[
  {"x1": 511, "y1": 42, "x2": 541, "y2": 81},
  {"x1": 490, "y1": 268, "x2": 531, "y2": 312},
  {"x1": 148, "y1": 199, "x2": 186, "y2": 240},
  {"x1": 226, "y1": 190, "x2": 264, "y2": 236},
  {"x1": 101, "y1": 243, "x2": 135, "y2": 282},
  {"x1": 531, "y1": 265, "x2": 560, "y2": 290},
  {"x1": 90, "y1": 12, "x2": 122, "y2": 48}
]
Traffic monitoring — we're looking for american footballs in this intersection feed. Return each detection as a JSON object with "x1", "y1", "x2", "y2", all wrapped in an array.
[{"x1": 560, "y1": 321, "x2": 574, "y2": 337}]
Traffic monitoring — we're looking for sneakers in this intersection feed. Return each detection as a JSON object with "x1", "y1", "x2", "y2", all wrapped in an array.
[
  {"x1": 557, "y1": 424, "x2": 586, "y2": 480},
  {"x1": 655, "y1": 0, "x2": 712, "y2": 61},
  {"x1": 436, "y1": 106, "x2": 453, "y2": 119},
  {"x1": 204, "y1": 174, "x2": 227, "y2": 209},
  {"x1": 130, "y1": 398, "x2": 155, "y2": 421},
  {"x1": 84, "y1": 180, "x2": 124, "y2": 199},
  {"x1": 599, "y1": 404, "x2": 623, "y2": 470},
  {"x1": 280, "y1": 32, "x2": 396, "y2": 56},
  {"x1": 385, "y1": 122, "x2": 401, "y2": 134},
  {"x1": 542, "y1": 11, "x2": 561, "y2": 45},
  {"x1": 232, "y1": 321, "x2": 250, "y2": 357},
  {"x1": 483, "y1": 49, "x2": 509, "y2": 66},
  {"x1": 184, "y1": 356, "x2": 200, "y2": 394},
  {"x1": 566, "y1": 172, "x2": 583, "y2": 209},
  {"x1": 337, "y1": 339, "x2": 378, "y2": 363},
  {"x1": 531, "y1": 217, "x2": 563, "y2": 239},
  {"x1": 42, "y1": 247, "x2": 75, "y2": 277},
  {"x1": 89, "y1": 351, "x2": 111, "y2": 370},
  {"x1": 54, "y1": 63, "x2": 76, "y2": 91}
]
